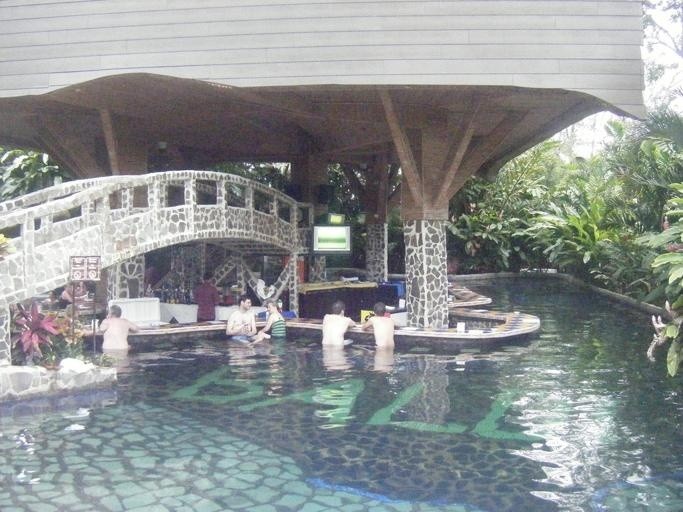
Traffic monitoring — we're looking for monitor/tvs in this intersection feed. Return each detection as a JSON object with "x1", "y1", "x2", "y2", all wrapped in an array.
[{"x1": 311, "y1": 224, "x2": 353, "y2": 254}]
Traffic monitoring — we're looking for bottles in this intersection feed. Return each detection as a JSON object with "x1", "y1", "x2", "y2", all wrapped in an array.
[{"x1": 145, "y1": 283, "x2": 193, "y2": 304}]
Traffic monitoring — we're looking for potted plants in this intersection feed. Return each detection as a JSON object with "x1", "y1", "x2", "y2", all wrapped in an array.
[{"x1": 10, "y1": 302, "x2": 84, "y2": 370}]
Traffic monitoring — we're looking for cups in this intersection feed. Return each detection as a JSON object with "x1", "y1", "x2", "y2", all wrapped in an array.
[{"x1": 457, "y1": 321, "x2": 466, "y2": 334}]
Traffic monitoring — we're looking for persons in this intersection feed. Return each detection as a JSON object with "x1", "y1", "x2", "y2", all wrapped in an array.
[
  {"x1": 195, "y1": 271, "x2": 220, "y2": 323},
  {"x1": 224, "y1": 296, "x2": 287, "y2": 347},
  {"x1": 360, "y1": 301, "x2": 396, "y2": 348},
  {"x1": 322, "y1": 301, "x2": 355, "y2": 344},
  {"x1": 40, "y1": 281, "x2": 87, "y2": 312},
  {"x1": 99, "y1": 304, "x2": 137, "y2": 360}
]
